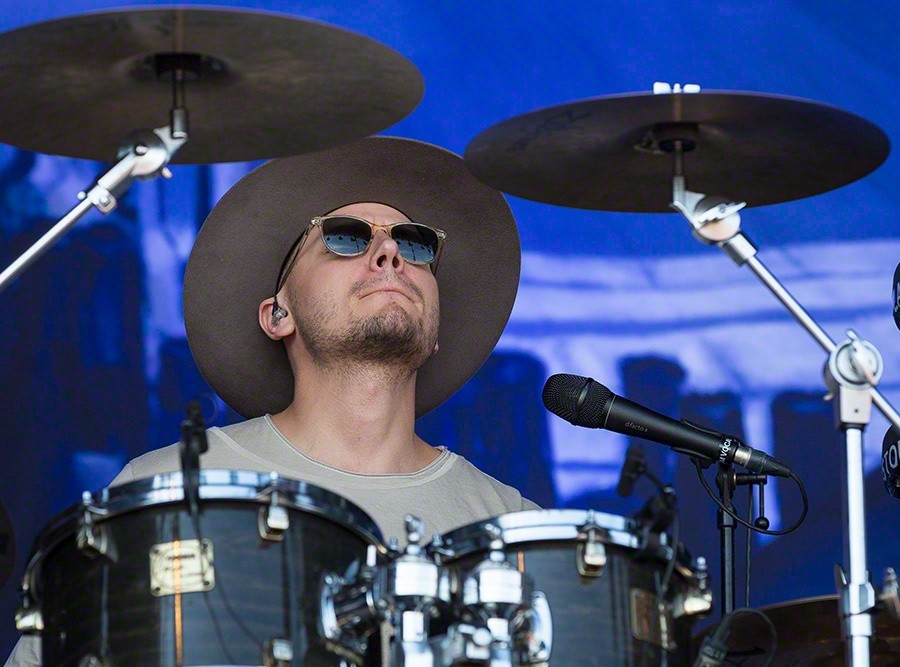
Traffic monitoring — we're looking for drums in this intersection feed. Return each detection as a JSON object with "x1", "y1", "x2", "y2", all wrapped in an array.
[
  {"x1": 28, "y1": 467, "x2": 403, "y2": 667},
  {"x1": 412, "y1": 510, "x2": 712, "y2": 667}
]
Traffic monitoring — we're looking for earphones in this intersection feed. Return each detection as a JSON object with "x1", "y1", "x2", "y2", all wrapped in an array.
[{"x1": 271, "y1": 309, "x2": 287, "y2": 325}]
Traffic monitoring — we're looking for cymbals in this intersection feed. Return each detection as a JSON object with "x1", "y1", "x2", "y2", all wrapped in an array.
[
  {"x1": 0, "y1": 6, "x2": 426, "y2": 165},
  {"x1": 463, "y1": 90, "x2": 893, "y2": 214}
]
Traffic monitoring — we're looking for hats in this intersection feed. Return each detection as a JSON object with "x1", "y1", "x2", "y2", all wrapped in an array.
[{"x1": 183, "y1": 137, "x2": 522, "y2": 419}]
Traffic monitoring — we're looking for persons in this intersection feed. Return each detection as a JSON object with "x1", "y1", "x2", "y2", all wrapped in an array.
[{"x1": 0, "y1": 136, "x2": 541, "y2": 667}]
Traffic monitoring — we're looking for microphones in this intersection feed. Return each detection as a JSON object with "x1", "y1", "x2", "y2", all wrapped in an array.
[
  {"x1": 187, "y1": 401, "x2": 208, "y2": 454},
  {"x1": 695, "y1": 612, "x2": 736, "y2": 667},
  {"x1": 618, "y1": 446, "x2": 643, "y2": 498},
  {"x1": 542, "y1": 374, "x2": 790, "y2": 475}
]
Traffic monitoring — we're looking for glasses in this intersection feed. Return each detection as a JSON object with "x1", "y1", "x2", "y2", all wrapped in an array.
[{"x1": 278, "y1": 214, "x2": 447, "y2": 292}]
